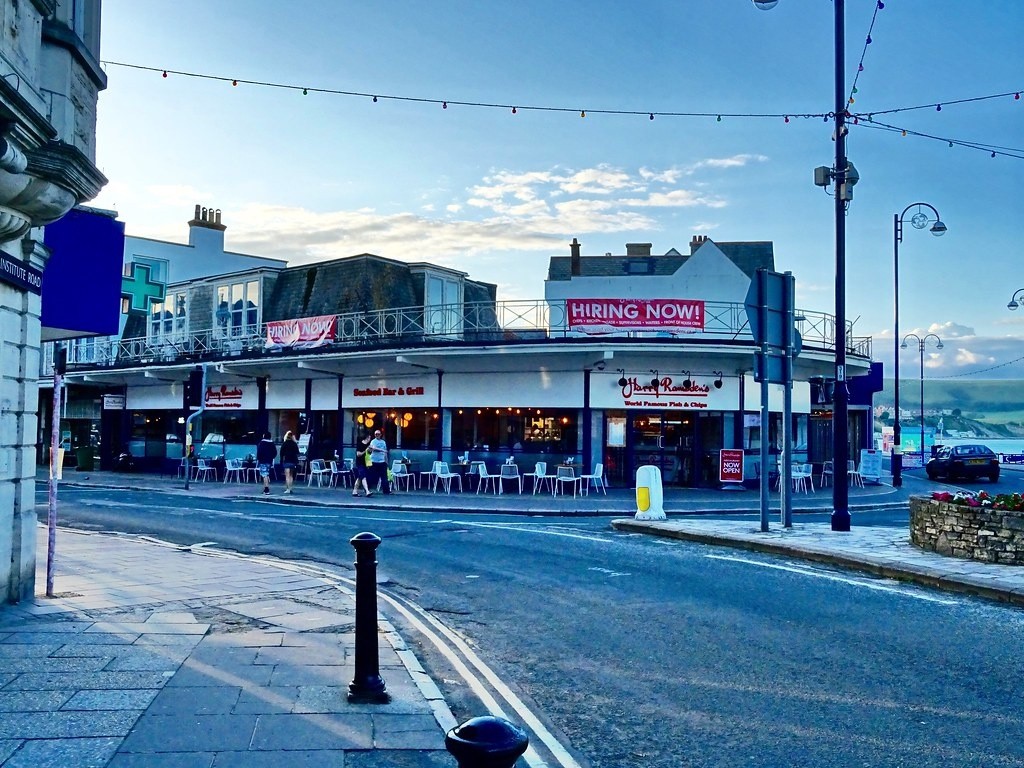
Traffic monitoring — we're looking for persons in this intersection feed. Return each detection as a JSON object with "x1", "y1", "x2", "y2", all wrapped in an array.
[
  {"x1": 113, "y1": 449, "x2": 134, "y2": 472},
  {"x1": 257, "y1": 431, "x2": 277, "y2": 495},
  {"x1": 368, "y1": 430, "x2": 395, "y2": 494},
  {"x1": 280, "y1": 432, "x2": 299, "y2": 494},
  {"x1": 352, "y1": 432, "x2": 374, "y2": 497}
]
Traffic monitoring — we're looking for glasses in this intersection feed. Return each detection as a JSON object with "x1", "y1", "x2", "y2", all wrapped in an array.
[{"x1": 375, "y1": 434, "x2": 380, "y2": 436}]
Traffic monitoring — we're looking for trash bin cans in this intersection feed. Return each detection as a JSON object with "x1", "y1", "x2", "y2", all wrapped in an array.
[
  {"x1": 634, "y1": 465, "x2": 666, "y2": 521},
  {"x1": 73, "y1": 445, "x2": 95, "y2": 471}
]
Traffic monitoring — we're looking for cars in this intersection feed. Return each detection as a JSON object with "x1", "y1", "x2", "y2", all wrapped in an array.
[{"x1": 926, "y1": 445, "x2": 1001, "y2": 484}]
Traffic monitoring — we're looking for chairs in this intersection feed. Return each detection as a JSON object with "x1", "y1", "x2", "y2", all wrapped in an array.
[
  {"x1": 177, "y1": 454, "x2": 279, "y2": 485},
  {"x1": 388, "y1": 459, "x2": 608, "y2": 500},
  {"x1": 774, "y1": 460, "x2": 815, "y2": 493},
  {"x1": 820, "y1": 459, "x2": 865, "y2": 490},
  {"x1": 308, "y1": 458, "x2": 357, "y2": 490}
]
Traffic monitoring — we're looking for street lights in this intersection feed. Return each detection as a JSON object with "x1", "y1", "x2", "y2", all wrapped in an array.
[
  {"x1": 893, "y1": 202, "x2": 947, "y2": 487},
  {"x1": 900, "y1": 334, "x2": 944, "y2": 464}
]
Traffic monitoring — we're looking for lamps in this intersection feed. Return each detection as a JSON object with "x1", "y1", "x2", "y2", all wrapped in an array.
[
  {"x1": 616, "y1": 368, "x2": 628, "y2": 388},
  {"x1": 713, "y1": 371, "x2": 723, "y2": 389},
  {"x1": 682, "y1": 370, "x2": 692, "y2": 389},
  {"x1": 357, "y1": 411, "x2": 377, "y2": 428},
  {"x1": 386, "y1": 411, "x2": 413, "y2": 428},
  {"x1": 650, "y1": 369, "x2": 660, "y2": 387}
]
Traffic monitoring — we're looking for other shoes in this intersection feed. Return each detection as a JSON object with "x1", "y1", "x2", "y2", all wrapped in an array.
[
  {"x1": 366, "y1": 492, "x2": 372, "y2": 496},
  {"x1": 283, "y1": 489, "x2": 291, "y2": 493},
  {"x1": 262, "y1": 489, "x2": 270, "y2": 494},
  {"x1": 386, "y1": 492, "x2": 394, "y2": 495},
  {"x1": 353, "y1": 493, "x2": 362, "y2": 497}
]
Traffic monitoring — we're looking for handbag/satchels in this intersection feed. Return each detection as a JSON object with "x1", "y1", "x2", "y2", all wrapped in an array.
[
  {"x1": 365, "y1": 449, "x2": 372, "y2": 466},
  {"x1": 387, "y1": 467, "x2": 394, "y2": 482}
]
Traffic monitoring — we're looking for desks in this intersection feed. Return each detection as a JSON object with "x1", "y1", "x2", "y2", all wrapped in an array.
[
  {"x1": 240, "y1": 459, "x2": 256, "y2": 482},
  {"x1": 448, "y1": 463, "x2": 484, "y2": 490},
  {"x1": 554, "y1": 463, "x2": 585, "y2": 469},
  {"x1": 401, "y1": 460, "x2": 422, "y2": 465}
]
